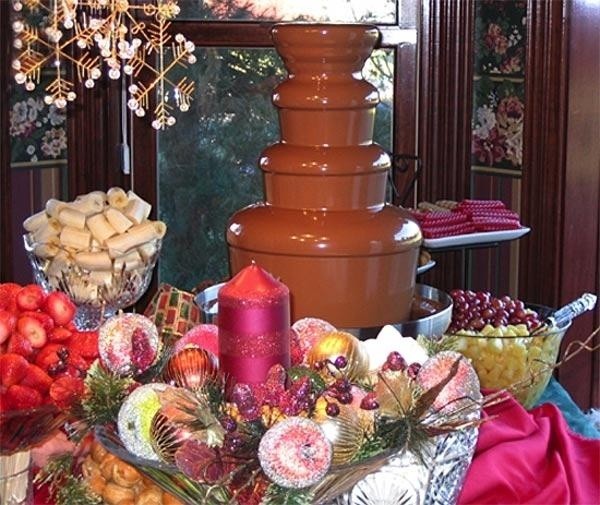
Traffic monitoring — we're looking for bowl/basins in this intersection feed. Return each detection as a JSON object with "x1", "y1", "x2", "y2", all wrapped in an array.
[
  {"x1": 24, "y1": 237, "x2": 165, "y2": 332},
  {"x1": 437, "y1": 301, "x2": 573, "y2": 411}
]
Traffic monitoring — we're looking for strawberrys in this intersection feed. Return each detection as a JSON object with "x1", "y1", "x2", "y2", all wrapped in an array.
[{"x1": 0, "y1": 283, "x2": 97, "y2": 414}]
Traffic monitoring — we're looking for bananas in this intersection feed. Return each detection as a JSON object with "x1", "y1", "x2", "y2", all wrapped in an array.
[{"x1": 22, "y1": 185, "x2": 166, "y2": 304}]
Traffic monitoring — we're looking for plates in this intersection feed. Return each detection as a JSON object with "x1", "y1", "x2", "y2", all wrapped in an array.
[{"x1": 424, "y1": 225, "x2": 532, "y2": 248}]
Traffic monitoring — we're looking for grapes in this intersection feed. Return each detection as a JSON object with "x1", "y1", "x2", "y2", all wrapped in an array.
[{"x1": 447, "y1": 289, "x2": 553, "y2": 334}]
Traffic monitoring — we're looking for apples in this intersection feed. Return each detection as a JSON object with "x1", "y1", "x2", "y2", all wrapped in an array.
[
  {"x1": 174, "y1": 324, "x2": 219, "y2": 357},
  {"x1": 290, "y1": 317, "x2": 337, "y2": 365},
  {"x1": 98, "y1": 313, "x2": 159, "y2": 375},
  {"x1": 257, "y1": 416, "x2": 332, "y2": 489},
  {"x1": 118, "y1": 383, "x2": 176, "y2": 460}
]
